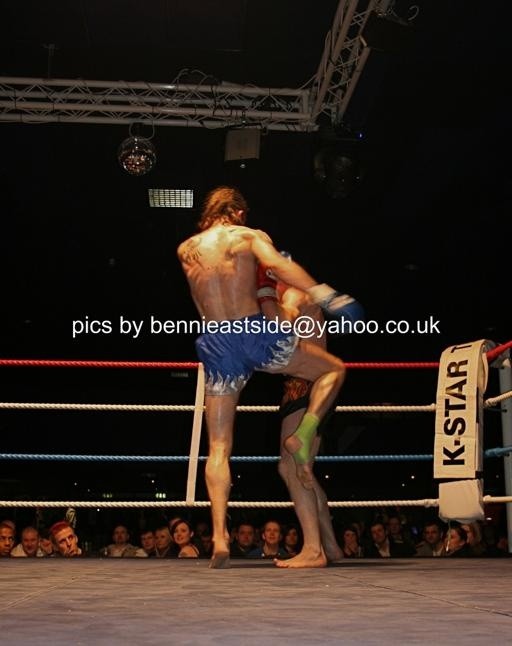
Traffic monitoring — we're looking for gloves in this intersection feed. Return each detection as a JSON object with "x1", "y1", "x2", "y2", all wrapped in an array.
[
  {"x1": 306, "y1": 283, "x2": 366, "y2": 334},
  {"x1": 254, "y1": 258, "x2": 279, "y2": 307}
]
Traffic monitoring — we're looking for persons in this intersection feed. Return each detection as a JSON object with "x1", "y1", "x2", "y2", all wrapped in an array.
[
  {"x1": 1, "y1": 489, "x2": 510, "y2": 558},
  {"x1": 252, "y1": 247, "x2": 346, "y2": 570},
  {"x1": 176, "y1": 185, "x2": 369, "y2": 568}
]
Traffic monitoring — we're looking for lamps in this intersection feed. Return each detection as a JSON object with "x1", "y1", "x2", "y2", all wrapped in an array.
[{"x1": 114, "y1": 126, "x2": 158, "y2": 179}]
